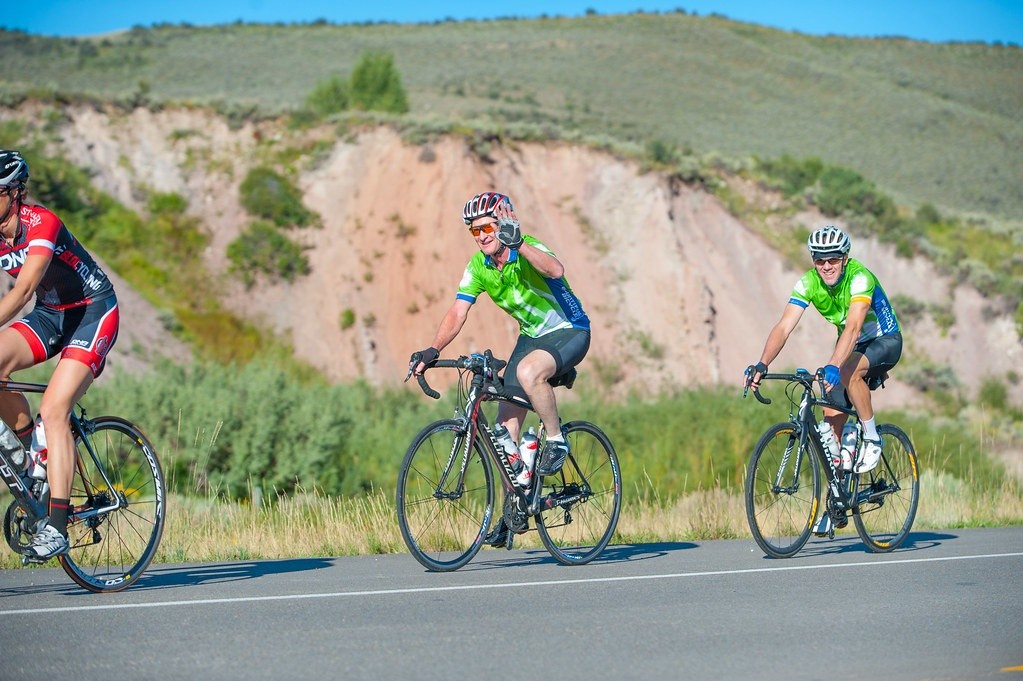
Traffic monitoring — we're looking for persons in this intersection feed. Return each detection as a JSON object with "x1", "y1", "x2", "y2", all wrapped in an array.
[
  {"x1": 407, "y1": 192, "x2": 592, "y2": 544},
  {"x1": 0, "y1": 148, "x2": 119, "y2": 559},
  {"x1": 741, "y1": 225, "x2": 902, "y2": 532}
]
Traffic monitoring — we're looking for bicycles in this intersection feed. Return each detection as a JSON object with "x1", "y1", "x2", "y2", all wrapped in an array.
[
  {"x1": 743, "y1": 365, "x2": 920, "y2": 559},
  {"x1": 0, "y1": 357, "x2": 165, "y2": 593},
  {"x1": 396, "y1": 350, "x2": 622, "y2": 572}
]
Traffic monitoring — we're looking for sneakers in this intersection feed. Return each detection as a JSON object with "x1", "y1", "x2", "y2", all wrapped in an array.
[
  {"x1": 812, "y1": 508, "x2": 849, "y2": 535},
  {"x1": 24, "y1": 523, "x2": 69, "y2": 560},
  {"x1": 538, "y1": 425, "x2": 569, "y2": 475},
  {"x1": 853, "y1": 430, "x2": 883, "y2": 474},
  {"x1": 484, "y1": 514, "x2": 529, "y2": 545},
  {"x1": 17, "y1": 470, "x2": 33, "y2": 491}
]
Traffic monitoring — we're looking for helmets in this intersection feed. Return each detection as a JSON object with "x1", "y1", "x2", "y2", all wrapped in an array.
[
  {"x1": 463, "y1": 192, "x2": 513, "y2": 225},
  {"x1": 0, "y1": 148, "x2": 29, "y2": 191},
  {"x1": 807, "y1": 225, "x2": 850, "y2": 258}
]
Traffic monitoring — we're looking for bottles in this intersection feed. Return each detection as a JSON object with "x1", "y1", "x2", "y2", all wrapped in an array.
[
  {"x1": 0, "y1": 418, "x2": 31, "y2": 472},
  {"x1": 818, "y1": 420, "x2": 841, "y2": 469},
  {"x1": 513, "y1": 426, "x2": 539, "y2": 487},
  {"x1": 493, "y1": 423, "x2": 532, "y2": 485},
  {"x1": 840, "y1": 418, "x2": 858, "y2": 473},
  {"x1": 28, "y1": 414, "x2": 50, "y2": 480}
]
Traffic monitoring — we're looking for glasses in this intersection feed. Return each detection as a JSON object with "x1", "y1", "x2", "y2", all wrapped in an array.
[
  {"x1": 468, "y1": 221, "x2": 500, "y2": 236},
  {"x1": 814, "y1": 259, "x2": 843, "y2": 265}
]
